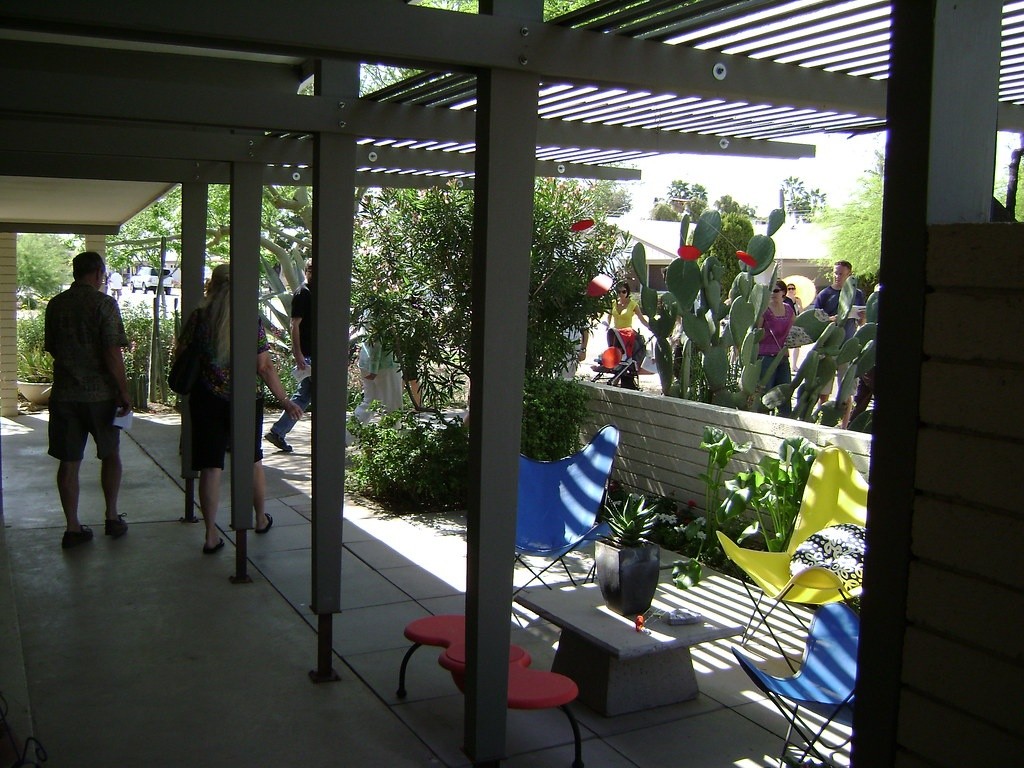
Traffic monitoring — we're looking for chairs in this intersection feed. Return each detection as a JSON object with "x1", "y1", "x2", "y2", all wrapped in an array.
[
  {"x1": 513, "y1": 424, "x2": 622, "y2": 598},
  {"x1": 716, "y1": 445, "x2": 869, "y2": 768}
]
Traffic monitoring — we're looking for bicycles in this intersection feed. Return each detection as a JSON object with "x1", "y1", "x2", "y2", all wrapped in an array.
[{"x1": 109, "y1": 281, "x2": 119, "y2": 301}]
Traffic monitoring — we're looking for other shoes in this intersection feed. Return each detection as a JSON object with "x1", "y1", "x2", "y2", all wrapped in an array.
[{"x1": 265, "y1": 433, "x2": 293, "y2": 452}]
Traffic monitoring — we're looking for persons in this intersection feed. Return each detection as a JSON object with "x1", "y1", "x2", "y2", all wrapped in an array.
[
  {"x1": 759, "y1": 278, "x2": 802, "y2": 418},
  {"x1": 344, "y1": 268, "x2": 424, "y2": 447},
  {"x1": 173, "y1": 264, "x2": 303, "y2": 555},
  {"x1": 590, "y1": 346, "x2": 627, "y2": 373},
  {"x1": 606, "y1": 280, "x2": 652, "y2": 332},
  {"x1": 264, "y1": 265, "x2": 313, "y2": 451},
  {"x1": 552, "y1": 318, "x2": 589, "y2": 378},
  {"x1": 661, "y1": 266, "x2": 700, "y2": 380},
  {"x1": 44, "y1": 252, "x2": 133, "y2": 548},
  {"x1": 814, "y1": 260, "x2": 880, "y2": 430},
  {"x1": 106, "y1": 271, "x2": 123, "y2": 303}
]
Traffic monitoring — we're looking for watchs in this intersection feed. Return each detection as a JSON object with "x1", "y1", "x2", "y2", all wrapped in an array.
[{"x1": 580, "y1": 348, "x2": 586, "y2": 353}]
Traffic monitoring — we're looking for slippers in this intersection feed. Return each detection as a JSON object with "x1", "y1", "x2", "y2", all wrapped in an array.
[
  {"x1": 255, "y1": 512, "x2": 272, "y2": 533},
  {"x1": 203, "y1": 536, "x2": 224, "y2": 553}
]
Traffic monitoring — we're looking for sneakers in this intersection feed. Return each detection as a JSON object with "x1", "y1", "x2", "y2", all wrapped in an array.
[
  {"x1": 62, "y1": 525, "x2": 92, "y2": 549},
  {"x1": 105, "y1": 512, "x2": 128, "y2": 535}
]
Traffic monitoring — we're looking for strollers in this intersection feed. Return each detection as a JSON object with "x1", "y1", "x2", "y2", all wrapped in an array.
[{"x1": 591, "y1": 326, "x2": 655, "y2": 392}]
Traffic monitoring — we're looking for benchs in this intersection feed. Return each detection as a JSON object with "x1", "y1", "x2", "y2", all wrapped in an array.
[{"x1": 396, "y1": 615, "x2": 585, "y2": 768}]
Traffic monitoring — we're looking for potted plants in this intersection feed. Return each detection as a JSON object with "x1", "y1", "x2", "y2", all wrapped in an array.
[
  {"x1": 18, "y1": 343, "x2": 56, "y2": 405},
  {"x1": 595, "y1": 494, "x2": 669, "y2": 616}
]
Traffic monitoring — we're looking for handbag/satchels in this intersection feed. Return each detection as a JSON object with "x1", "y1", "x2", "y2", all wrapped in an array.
[
  {"x1": 640, "y1": 339, "x2": 658, "y2": 373},
  {"x1": 168, "y1": 308, "x2": 203, "y2": 395}
]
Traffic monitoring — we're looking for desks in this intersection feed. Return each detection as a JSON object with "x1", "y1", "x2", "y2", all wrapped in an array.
[{"x1": 513, "y1": 580, "x2": 746, "y2": 718}]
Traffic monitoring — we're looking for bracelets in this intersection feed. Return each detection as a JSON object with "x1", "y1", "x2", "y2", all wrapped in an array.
[{"x1": 279, "y1": 396, "x2": 288, "y2": 402}]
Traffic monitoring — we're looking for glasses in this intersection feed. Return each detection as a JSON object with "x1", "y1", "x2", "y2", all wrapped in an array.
[
  {"x1": 788, "y1": 288, "x2": 795, "y2": 290},
  {"x1": 773, "y1": 289, "x2": 782, "y2": 293},
  {"x1": 617, "y1": 290, "x2": 627, "y2": 295}
]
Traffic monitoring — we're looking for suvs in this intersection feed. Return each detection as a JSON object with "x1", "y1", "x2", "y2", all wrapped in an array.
[{"x1": 130, "y1": 266, "x2": 175, "y2": 295}]
